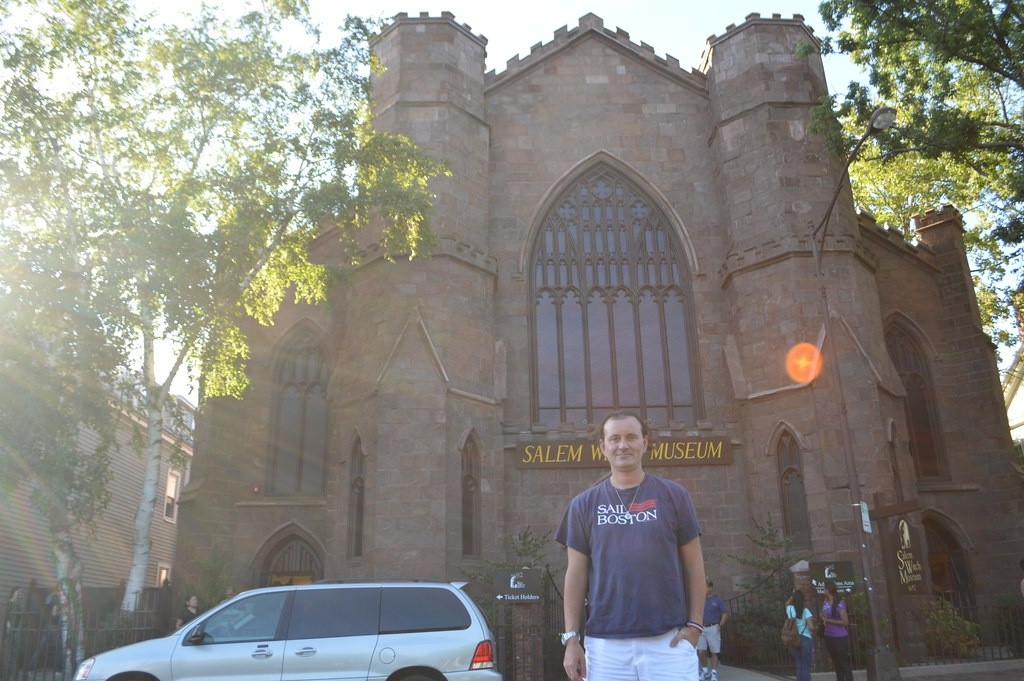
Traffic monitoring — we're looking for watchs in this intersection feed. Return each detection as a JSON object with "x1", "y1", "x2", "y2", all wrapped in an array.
[{"x1": 561, "y1": 632, "x2": 579, "y2": 645}]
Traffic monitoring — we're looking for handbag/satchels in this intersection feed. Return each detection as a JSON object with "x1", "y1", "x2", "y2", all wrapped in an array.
[{"x1": 817, "y1": 621, "x2": 825, "y2": 638}]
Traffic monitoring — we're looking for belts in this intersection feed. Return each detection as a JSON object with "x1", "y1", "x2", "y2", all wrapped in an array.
[{"x1": 704, "y1": 623, "x2": 711, "y2": 627}]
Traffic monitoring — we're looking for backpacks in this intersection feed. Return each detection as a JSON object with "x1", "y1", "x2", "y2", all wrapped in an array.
[{"x1": 781, "y1": 604, "x2": 807, "y2": 649}]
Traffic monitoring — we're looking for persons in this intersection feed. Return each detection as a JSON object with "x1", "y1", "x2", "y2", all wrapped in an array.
[
  {"x1": 175, "y1": 595, "x2": 198, "y2": 630},
  {"x1": 785, "y1": 590, "x2": 816, "y2": 681},
  {"x1": 697, "y1": 577, "x2": 729, "y2": 681},
  {"x1": 818, "y1": 580, "x2": 854, "y2": 681},
  {"x1": 555, "y1": 410, "x2": 706, "y2": 681},
  {"x1": 6, "y1": 586, "x2": 22, "y2": 633},
  {"x1": 27, "y1": 601, "x2": 60, "y2": 677},
  {"x1": 221, "y1": 586, "x2": 236, "y2": 603}
]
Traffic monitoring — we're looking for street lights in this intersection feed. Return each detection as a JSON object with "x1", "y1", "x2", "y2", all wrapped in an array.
[{"x1": 804, "y1": 101, "x2": 905, "y2": 681}]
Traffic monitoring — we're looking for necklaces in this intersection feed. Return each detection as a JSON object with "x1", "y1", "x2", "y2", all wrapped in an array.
[{"x1": 614, "y1": 481, "x2": 641, "y2": 520}]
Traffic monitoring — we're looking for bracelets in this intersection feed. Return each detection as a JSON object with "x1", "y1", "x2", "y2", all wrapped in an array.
[
  {"x1": 686, "y1": 622, "x2": 704, "y2": 633},
  {"x1": 718, "y1": 624, "x2": 720, "y2": 626}
]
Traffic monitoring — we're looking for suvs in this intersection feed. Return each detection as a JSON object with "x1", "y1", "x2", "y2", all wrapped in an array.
[{"x1": 69, "y1": 579, "x2": 503, "y2": 681}]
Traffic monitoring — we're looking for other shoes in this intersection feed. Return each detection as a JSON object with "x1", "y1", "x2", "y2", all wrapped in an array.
[
  {"x1": 710, "y1": 671, "x2": 718, "y2": 681},
  {"x1": 699, "y1": 668, "x2": 710, "y2": 681}
]
